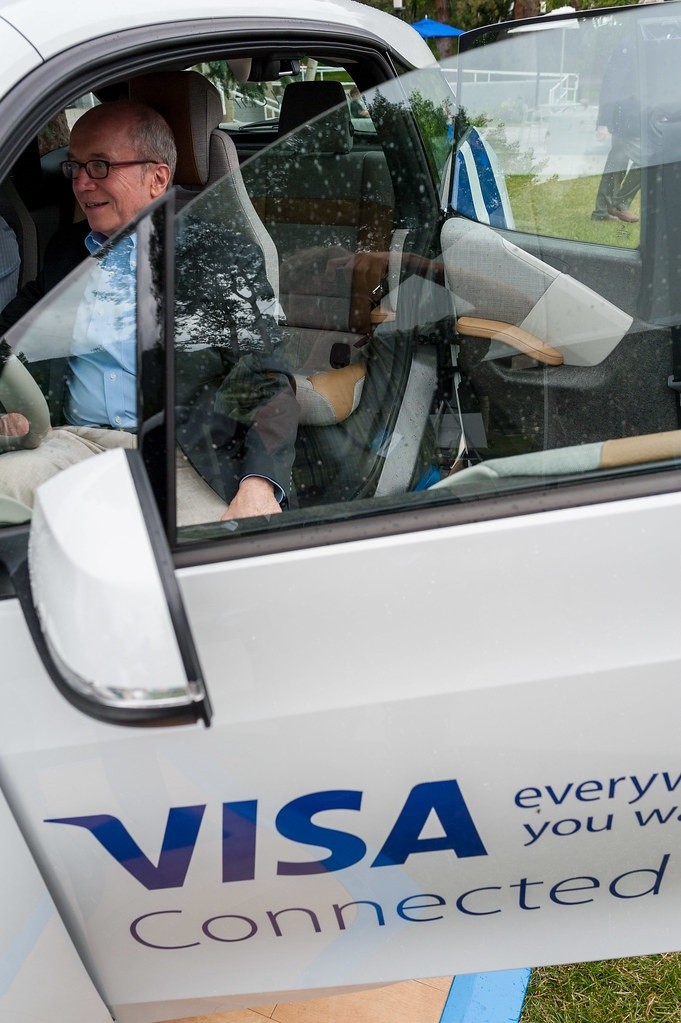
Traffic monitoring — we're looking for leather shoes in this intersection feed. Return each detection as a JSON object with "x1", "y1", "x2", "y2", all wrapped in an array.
[{"x1": 608, "y1": 204, "x2": 639, "y2": 223}]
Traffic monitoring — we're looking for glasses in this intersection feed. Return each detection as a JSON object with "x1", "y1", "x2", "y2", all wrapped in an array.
[{"x1": 58, "y1": 159, "x2": 158, "y2": 180}]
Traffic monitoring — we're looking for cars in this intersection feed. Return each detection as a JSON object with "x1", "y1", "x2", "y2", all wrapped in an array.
[{"x1": 0, "y1": 4, "x2": 681, "y2": 1022}]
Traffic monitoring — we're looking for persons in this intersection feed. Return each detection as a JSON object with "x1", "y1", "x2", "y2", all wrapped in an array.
[
  {"x1": 0, "y1": 102, "x2": 303, "y2": 526},
  {"x1": 351, "y1": 87, "x2": 370, "y2": 118},
  {"x1": 592, "y1": 40, "x2": 640, "y2": 224}
]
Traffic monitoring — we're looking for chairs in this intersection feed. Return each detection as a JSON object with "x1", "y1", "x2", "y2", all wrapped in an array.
[
  {"x1": 5, "y1": 135, "x2": 50, "y2": 296},
  {"x1": 244, "y1": 75, "x2": 395, "y2": 428},
  {"x1": 134, "y1": 66, "x2": 282, "y2": 326}
]
